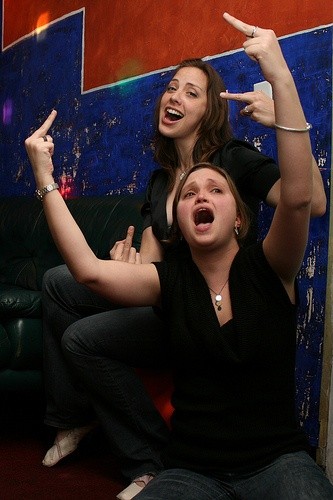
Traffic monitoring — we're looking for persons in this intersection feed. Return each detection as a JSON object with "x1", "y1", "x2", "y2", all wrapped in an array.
[{"x1": 24, "y1": 12, "x2": 332, "y2": 499}]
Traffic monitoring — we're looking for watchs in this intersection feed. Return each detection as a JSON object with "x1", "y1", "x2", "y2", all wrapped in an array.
[
  {"x1": 35, "y1": 182, "x2": 60, "y2": 201},
  {"x1": 40, "y1": 58, "x2": 327, "y2": 499}
]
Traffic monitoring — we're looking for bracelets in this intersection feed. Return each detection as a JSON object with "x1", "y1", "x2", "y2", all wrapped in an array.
[{"x1": 272, "y1": 122, "x2": 313, "y2": 132}]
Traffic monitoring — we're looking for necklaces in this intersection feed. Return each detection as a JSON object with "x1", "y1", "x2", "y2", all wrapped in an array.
[
  {"x1": 208, "y1": 279, "x2": 230, "y2": 312},
  {"x1": 177, "y1": 164, "x2": 193, "y2": 180}
]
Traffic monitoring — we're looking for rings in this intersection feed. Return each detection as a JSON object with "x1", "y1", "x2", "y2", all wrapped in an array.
[
  {"x1": 251, "y1": 26, "x2": 259, "y2": 37},
  {"x1": 244, "y1": 106, "x2": 249, "y2": 112},
  {"x1": 41, "y1": 136, "x2": 48, "y2": 141}
]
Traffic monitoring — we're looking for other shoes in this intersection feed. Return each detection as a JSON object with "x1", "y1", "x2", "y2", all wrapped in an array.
[
  {"x1": 116, "y1": 481, "x2": 143, "y2": 500},
  {"x1": 42, "y1": 430, "x2": 78, "y2": 467}
]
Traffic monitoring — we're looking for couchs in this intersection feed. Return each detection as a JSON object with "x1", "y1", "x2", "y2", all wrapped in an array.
[{"x1": 0, "y1": 197, "x2": 145, "y2": 394}]
